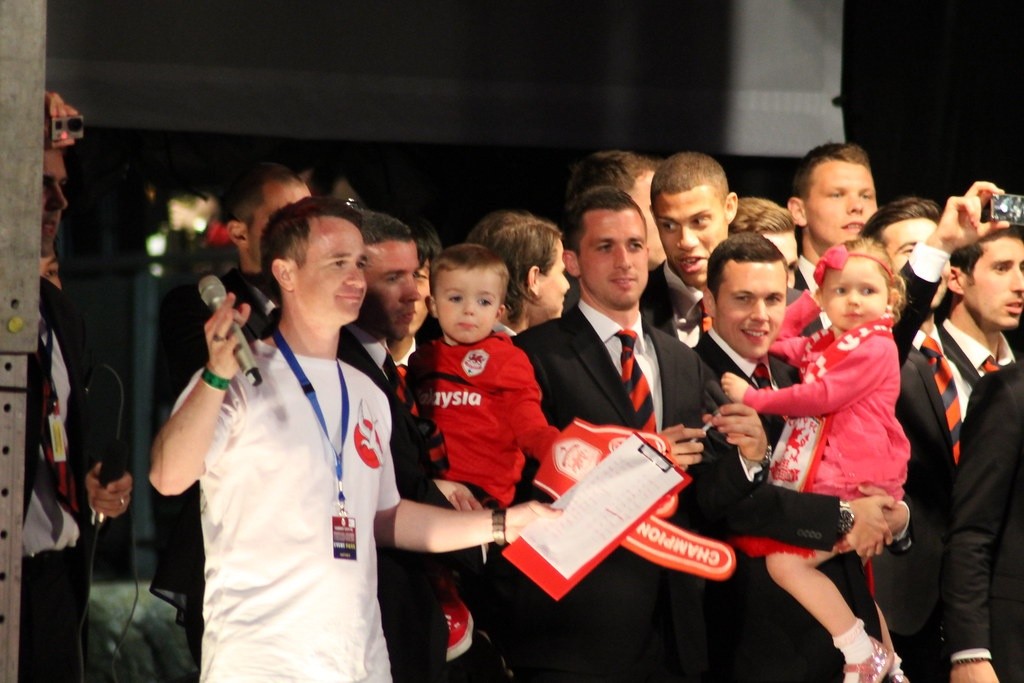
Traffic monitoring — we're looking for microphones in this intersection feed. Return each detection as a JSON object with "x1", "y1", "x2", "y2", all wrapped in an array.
[
  {"x1": 95, "y1": 437, "x2": 129, "y2": 529},
  {"x1": 197, "y1": 274, "x2": 263, "y2": 387}
]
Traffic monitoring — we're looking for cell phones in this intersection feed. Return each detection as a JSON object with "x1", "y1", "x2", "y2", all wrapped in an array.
[{"x1": 990, "y1": 193, "x2": 1024, "y2": 226}]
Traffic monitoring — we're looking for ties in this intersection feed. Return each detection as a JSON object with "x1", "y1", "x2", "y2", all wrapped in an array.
[
  {"x1": 382, "y1": 347, "x2": 449, "y2": 473},
  {"x1": 697, "y1": 298, "x2": 713, "y2": 334},
  {"x1": 615, "y1": 329, "x2": 657, "y2": 435},
  {"x1": 35, "y1": 335, "x2": 79, "y2": 514},
  {"x1": 750, "y1": 362, "x2": 788, "y2": 431},
  {"x1": 983, "y1": 358, "x2": 1005, "y2": 374},
  {"x1": 919, "y1": 335, "x2": 962, "y2": 466}
]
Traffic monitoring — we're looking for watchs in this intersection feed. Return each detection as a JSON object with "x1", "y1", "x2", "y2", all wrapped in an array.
[
  {"x1": 761, "y1": 445, "x2": 772, "y2": 467},
  {"x1": 837, "y1": 498, "x2": 854, "y2": 533}
]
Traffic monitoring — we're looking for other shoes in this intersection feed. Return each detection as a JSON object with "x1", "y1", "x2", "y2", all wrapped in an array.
[
  {"x1": 892, "y1": 674, "x2": 910, "y2": 683},
  {"x1": 843, "y1": 635, "x2": 894, "y2": 683}
]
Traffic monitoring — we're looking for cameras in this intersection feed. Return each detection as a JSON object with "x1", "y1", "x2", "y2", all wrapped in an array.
[{"x1": 51, "y1": 115, "x2": 84, "y2": 142}]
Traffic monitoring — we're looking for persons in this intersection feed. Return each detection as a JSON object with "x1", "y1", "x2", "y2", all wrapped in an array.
[
  {"x1": 1, "y1": 91, "x2": 132, "y2": 683},
  {"x1": 149, "y1": 143, "x2": 1024, "y2": 683}
]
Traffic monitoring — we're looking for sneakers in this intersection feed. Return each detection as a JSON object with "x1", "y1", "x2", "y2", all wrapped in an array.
[{"x1": 442, "y1": 600, "x2": 474, "y2": 663}]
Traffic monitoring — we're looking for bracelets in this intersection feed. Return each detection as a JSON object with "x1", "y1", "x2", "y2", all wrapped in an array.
[
  {"x1": 492, "y1": 509, "x2": 506, "y2": 545},
  {"x1": 201, "y1": 367, "x2": 231, "y2": 391}
]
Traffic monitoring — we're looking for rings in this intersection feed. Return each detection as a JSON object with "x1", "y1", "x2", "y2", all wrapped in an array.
[
  {"x1": 213, "y1": 334, "x2": 225, "y2": 341},
  {"x1": 120, "y1": 500, "x2": 124, "y2": 505}
]
